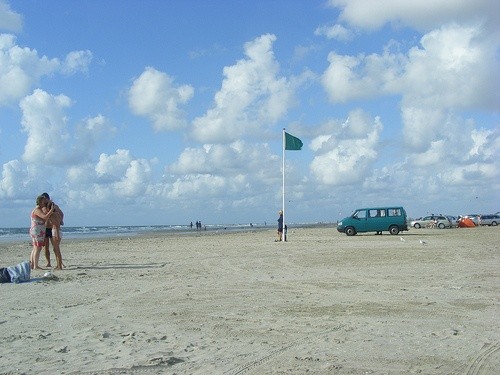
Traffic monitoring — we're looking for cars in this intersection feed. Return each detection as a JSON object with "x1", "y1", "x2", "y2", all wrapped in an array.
[
  {"x1": 411, "y1": 215, "x2": 450, "y2": 229},
  {"x1": 444, "y1": 215, "x2": 458, "y2": 227},
  {"x1": 465, "y1": 214, "x2": 478, "y2": 225},
  {"x1": 478, "y1": 214, "x2": 500, "y2": 226}
]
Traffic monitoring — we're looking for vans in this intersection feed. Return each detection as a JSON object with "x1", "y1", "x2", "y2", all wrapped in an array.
[{"x1": 336, "y1": 206, "x2": 409, "y2": 235}]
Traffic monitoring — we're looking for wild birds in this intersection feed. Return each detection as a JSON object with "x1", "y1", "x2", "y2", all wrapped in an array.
[
  {"x1": 400, "y1": 238, "x2": 405, "y2": 242},
  {"x1": 420, "y1": 240, "x2": 428, "y2": 245}
]
